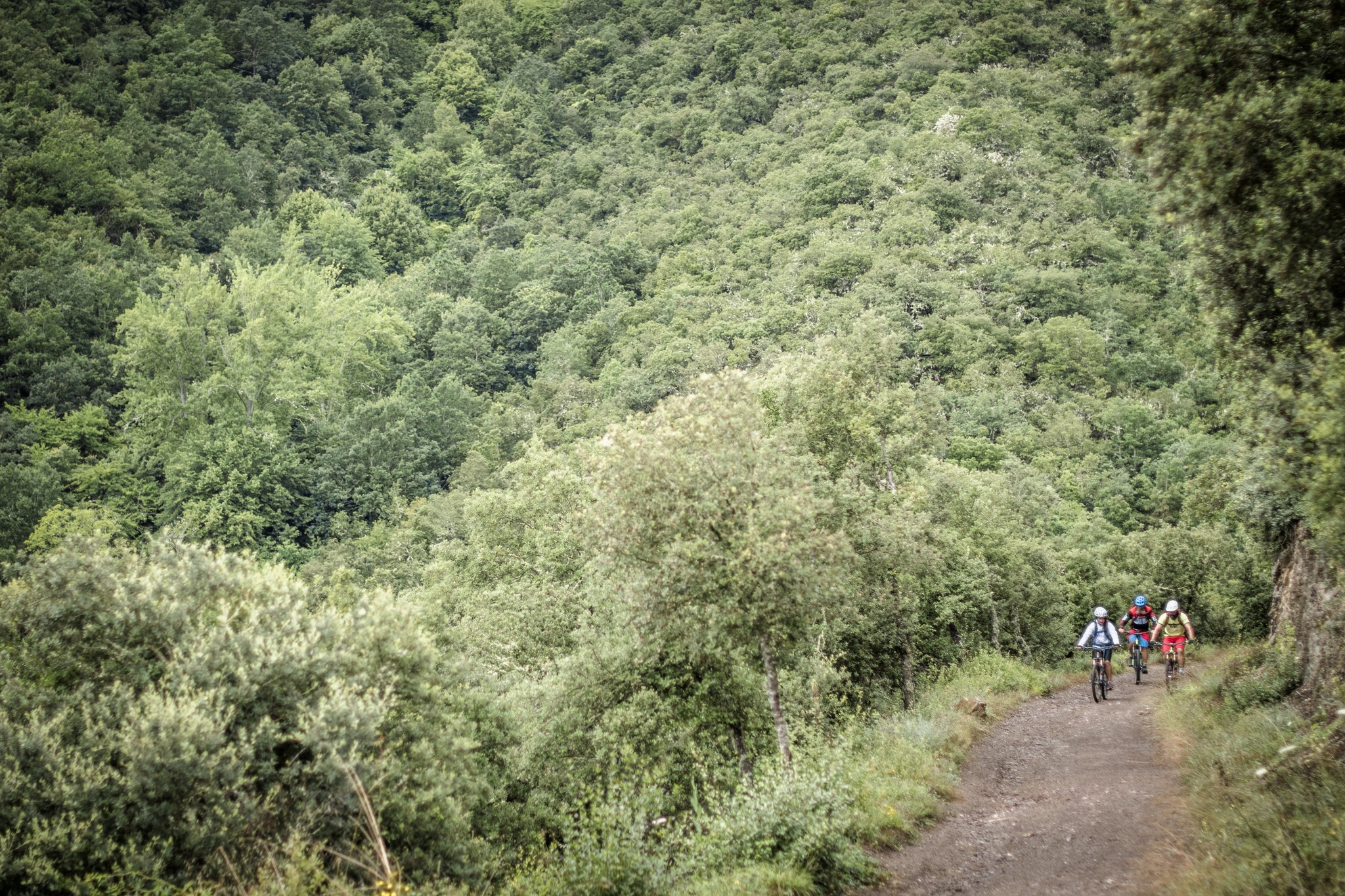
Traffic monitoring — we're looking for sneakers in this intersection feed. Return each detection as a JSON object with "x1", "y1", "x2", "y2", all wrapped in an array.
[
  {"x1": 1107, "y1": 683, "x2": 1114, "y2": 690},
  {"x1": 1142, "y1": 666, "x2": 1147, "y2": 673},
  {"x1": 1128, "y1": 657, "x2": 1133, "y2": 666},
  {"x1": 1088, "y1": 676, "x2": 1098, "y2": 682}
]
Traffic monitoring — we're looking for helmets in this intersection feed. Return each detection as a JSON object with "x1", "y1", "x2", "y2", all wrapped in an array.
[
  {"x1": 1166, "y1": 600, "x2": 1179, "y2": 611},
  {"x1": 1095, "y1": 607, "x2": 1107, "y2": 618},
  {"x1": 1135, "y1": 596, "x2": 1147, "y2": 606}
]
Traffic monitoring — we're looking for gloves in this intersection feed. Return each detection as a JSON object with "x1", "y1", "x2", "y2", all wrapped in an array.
[
  {"x1": 1188, "y1": 638, "x2": 1195, "y2": 644},
  {"x1": 1150, "y1": 640, "x2": 1158, "y2": 645}
]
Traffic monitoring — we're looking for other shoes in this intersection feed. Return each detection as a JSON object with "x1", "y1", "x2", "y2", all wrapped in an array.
[
  {"x1": 1179, "y1": 667, "x2": 1184, "y2": 675},
  {"x1": 1167, "y1": 666, "x2": 1172, "y2": 673}
]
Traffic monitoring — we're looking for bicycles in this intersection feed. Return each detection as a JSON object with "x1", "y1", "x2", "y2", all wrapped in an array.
[
  {"x1": 1074, "y1": 646, "x2": 1122, "y2": 703},
  {"x1": 1153, "y1": 640, "x2": 1192, "y2": 694},
  {"x1": 1122, "y1": 630, "x2": 1153, "y2": 685}
]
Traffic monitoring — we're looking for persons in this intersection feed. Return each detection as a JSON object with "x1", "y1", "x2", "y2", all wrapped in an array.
[
  {"x1": 1118, "y1": 596, "x2": 1158, "y2": 674},
  {"x1": 1077, "y1": 607, "x2": 1120, "y2": 689},
  {"x1": 1150, "y1": 600, "x2": 1194, "y2": 678}
]
